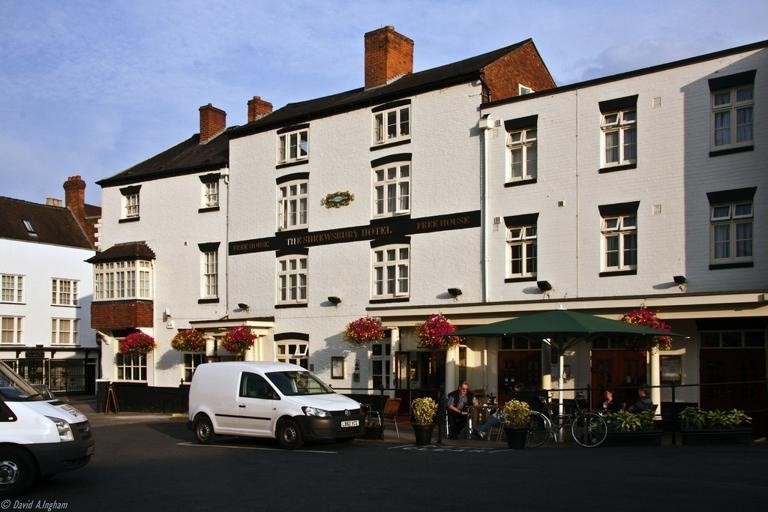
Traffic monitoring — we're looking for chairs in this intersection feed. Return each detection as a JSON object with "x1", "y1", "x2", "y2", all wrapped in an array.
[
  {"x1": 375, "y1": 397, "x2": 401, "y2": 439},
  {"x1": 539, "y1": 396, "x2": 663, "y2": 446},
  {"x1": 445, "y1": 402, "x2": 475, "y2": 439}
]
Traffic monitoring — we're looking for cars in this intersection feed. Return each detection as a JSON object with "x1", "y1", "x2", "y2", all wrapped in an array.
[{"x1": 29, "y1": 383, "x2": 54, "y2": 400}]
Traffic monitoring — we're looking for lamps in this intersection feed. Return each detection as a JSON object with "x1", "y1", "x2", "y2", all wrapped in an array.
[
  {"x1": 326, "y1": 297, "x2": 341, "y2": 307},
  {"x1": 237, "y1": 303, "x2": 249, "y2": 312},
  {"x1": 535, "y1": 280, "x2": 553, "y2": 301},
  {"x1": 672, "y1": 274, "x2": 688, "y2": 291},
  {"x1": 447, "y1": 288, "x2": 462, "y2": 298}
]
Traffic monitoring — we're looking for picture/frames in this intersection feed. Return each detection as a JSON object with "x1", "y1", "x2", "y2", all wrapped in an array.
[{"x1": 331, "y1": 356, "x2": 344, "y2": 379}]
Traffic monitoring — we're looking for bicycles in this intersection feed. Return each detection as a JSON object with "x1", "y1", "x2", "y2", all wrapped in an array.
[{"x1": 515, "y1": 391, "x2": 609, "y2": 449}]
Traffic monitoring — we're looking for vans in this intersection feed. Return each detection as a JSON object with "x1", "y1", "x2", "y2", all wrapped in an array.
[
  {"x1": 0, "y1": 359, "x2": 98, "y2": 493},
  {"x1": 187, "y1": 360, "x2": 365, "y2": 451}
]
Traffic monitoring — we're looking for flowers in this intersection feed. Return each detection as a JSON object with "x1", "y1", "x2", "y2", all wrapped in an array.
[
  {"x1": 221, "y1": 325, "x2": 259, "y2": 352},
  {"x1": 414, "y1": 310, "x2": 464, "y2": 350},
  {"x1": 341, "y1": 316, "x2": 385, "y2": 344},
  {"x1": 612, "y1": 306, "x2": 673, "y2": 352},
  {"x1": 170, "y1": 327, "x2": 207, "y2": 354},
  {"x1": 119, "y1": 330, "x2": 158, "y2": 356}
]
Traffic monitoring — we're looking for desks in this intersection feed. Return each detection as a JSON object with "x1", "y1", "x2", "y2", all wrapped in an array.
[{"x1": 463, "y1": 403, "x2": 504, "y2": 439}]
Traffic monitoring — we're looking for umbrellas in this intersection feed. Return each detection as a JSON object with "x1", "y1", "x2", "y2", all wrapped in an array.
[{"x1": 438, "y1": 311, "x2": 691, "y2": 445}]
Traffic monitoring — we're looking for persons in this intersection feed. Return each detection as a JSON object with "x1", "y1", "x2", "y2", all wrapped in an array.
[
  {"x1": 627, "y1": 383, "x2": 653, "y2": 415},
  {"x1": 446, "y1": 382, "x2": 478, "y2": 440},
  {"x1": 474, "y1": 384, "x2": 524, "y2": 439},
  {"x1": 596, "y1": 389, "x2": 620, "y2": 413}
]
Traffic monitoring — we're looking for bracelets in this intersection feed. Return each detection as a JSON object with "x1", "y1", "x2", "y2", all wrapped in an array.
[{"x1": 460, "y1": 411, "x2": 462, "y2": 415}]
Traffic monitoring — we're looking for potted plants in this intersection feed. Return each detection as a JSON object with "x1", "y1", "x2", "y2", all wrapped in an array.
[
  {"x1": 499, "y1": 396, "x2": 534, "y2": 450},
  {"x1": 408, "y1": 395, "x2": 439, "y2": 444},
  {"x1": 673, "y1": 405, "x2": 756, "y2": 446}
]
